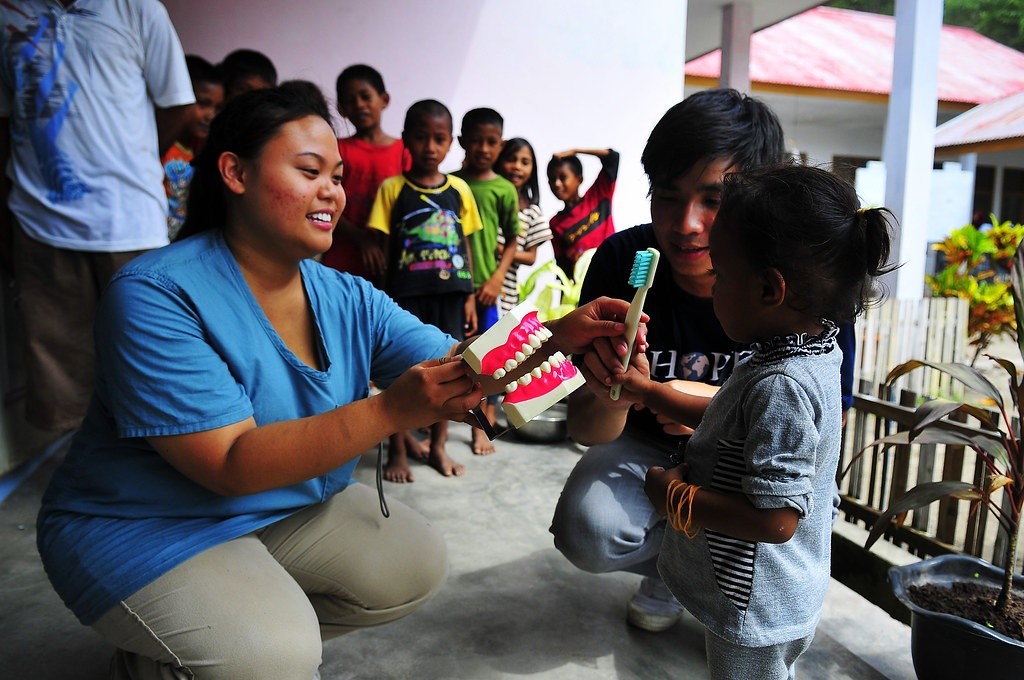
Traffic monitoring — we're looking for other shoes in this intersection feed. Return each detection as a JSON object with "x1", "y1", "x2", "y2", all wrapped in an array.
[{"x1": 626, "y1": 577, "x2": 683, "y2": 632}]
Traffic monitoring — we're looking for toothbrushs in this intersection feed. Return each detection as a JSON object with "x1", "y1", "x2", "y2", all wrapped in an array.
[{"x1": 608, "y1": 247, "x2": 660, "y2": 401}]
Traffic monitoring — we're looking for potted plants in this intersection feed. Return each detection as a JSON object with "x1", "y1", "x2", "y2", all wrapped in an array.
[{"x1": 841, "y1": 243, "x2": 1024, "y2": 680}]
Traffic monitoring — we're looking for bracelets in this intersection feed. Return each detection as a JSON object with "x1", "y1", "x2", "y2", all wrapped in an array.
[{"x1": 664, "y1": 478, "x2": 704, "y2": 540}]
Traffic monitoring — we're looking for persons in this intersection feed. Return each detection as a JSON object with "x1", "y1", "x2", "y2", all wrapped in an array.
[
  {"x1": 153, "y1": 48, "x2": 624, "y2": 487},
  {"x1": 1, "y1": 0, "x2": 195, "y2": 478},
  {"x1": 27, "y1": 78, "x2": 653, "y2": 680},
  {"x1": 605, "y1": 162, "x2": 889, "y2": 680},
  {"x1": 547, "y1": 86, "x2": 858, "y2": 632}
]
